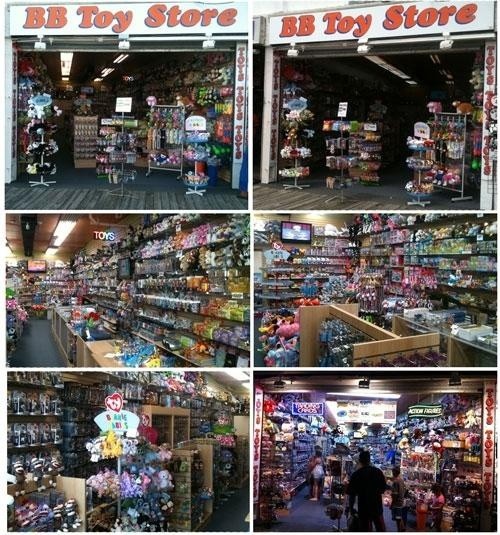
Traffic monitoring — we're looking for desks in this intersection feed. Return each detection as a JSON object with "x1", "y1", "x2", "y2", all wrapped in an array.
[{"x1": 391, "y1": 314, "x2": 497, "y2": 367}]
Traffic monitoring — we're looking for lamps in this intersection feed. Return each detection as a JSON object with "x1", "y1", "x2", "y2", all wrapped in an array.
[
  {"x1": 35, "y1": 36, "x2": 47, "y2": 50},
  {"x1": 448, "y1": 375, "x2": 462, "y2": 386},
  {"x1": 118, "y1": 35, "x2": 130, "y2": 49},
  {"x1": 273, "y1": 376, "x2": 286, "y2": 388},
  {"x1": 200, "y1": 33, "x2": 215, "y2": 49},
  {"x1": 359, "y1": 378, "x2": 369, "y2": 389},
  {"x1": 357, "y1": 38, "x2": 370, "y2": 53},
  {"x1": 287, "y1": 42, "x2": 299, "y2": 57},
  {"x1": 438, "y1": 31, "x2": 453, "y2": 50}
]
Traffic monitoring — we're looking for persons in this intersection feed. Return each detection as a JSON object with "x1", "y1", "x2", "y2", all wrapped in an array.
[
  {"x1": 304, "y1": 450, "x2": 326, "y2": 502},
  {"x1": 347, "y1": 447, "x2": 445, "y2": 532}
]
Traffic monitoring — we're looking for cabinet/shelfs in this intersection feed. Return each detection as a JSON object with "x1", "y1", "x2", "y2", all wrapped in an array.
[
  {"x1": 52, "y1": 209, "x2": 250, "y2": 367},
  {"x1": 140, "y1": 61, "x2": 234, "y2": 183},
  {"x1": 7, "y1": 372, "x2": 215, "y2": 532},
  {"x1": 272, "y1": 439, "x2": 312, "y2": 497},
  {"x1": 316, "y1": 90, "x2": 339, "y2": 121},
  {"x1": 258, "y1": 213, "x2": 497, "y2": 315}
]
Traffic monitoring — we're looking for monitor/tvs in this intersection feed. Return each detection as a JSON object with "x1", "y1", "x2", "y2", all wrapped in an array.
[
  {"x1": 117, "y1": 257, "x2": 132, "y2": 280},
  {"x1": 27, "y1": 260, "x2": 46, "y2": 273},
  {"x1": 281, "y1": 221, "x2": 311, "y2": 244}
]
